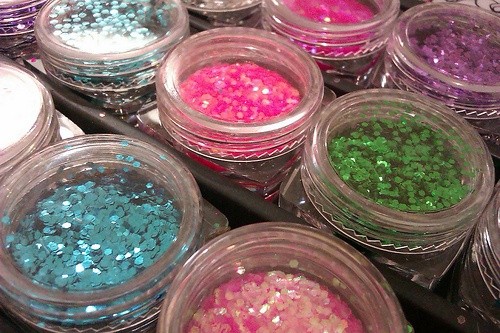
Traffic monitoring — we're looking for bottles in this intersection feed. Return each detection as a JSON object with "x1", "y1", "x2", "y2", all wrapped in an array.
[{"x1": 1, "y1": 0, "x2": 499, "y2": 332}]
